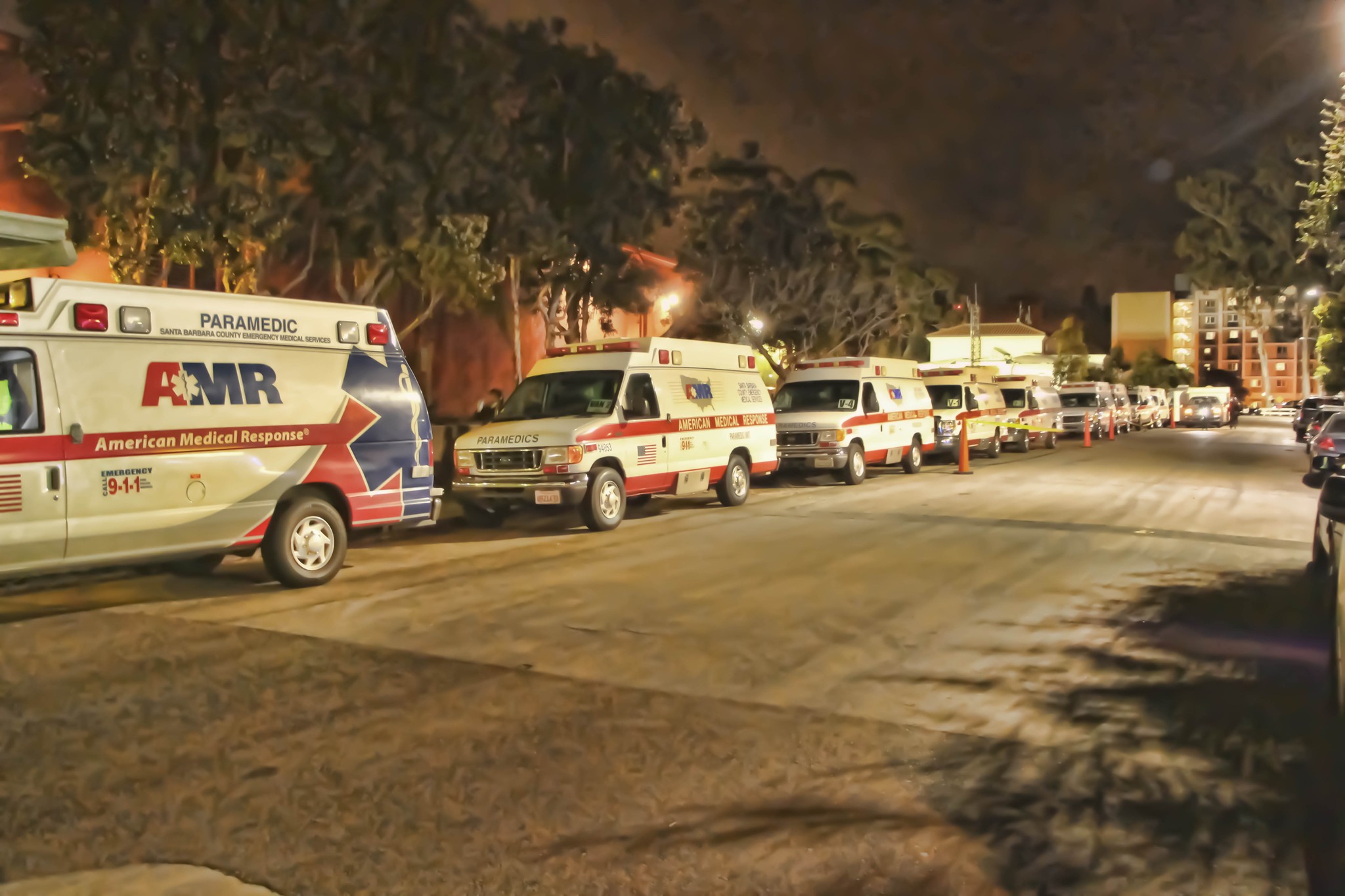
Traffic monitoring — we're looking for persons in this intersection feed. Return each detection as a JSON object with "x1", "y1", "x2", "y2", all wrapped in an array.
[
  {"x1": 1227, "y1": 394, "x2": 1241, "y2": 428},
  {"x1": 1199, "y1": 395, "x2": 1211, "y2": 430},
  {"x1": 477, "y1": 388, "x2": 504, "y2": 419}
]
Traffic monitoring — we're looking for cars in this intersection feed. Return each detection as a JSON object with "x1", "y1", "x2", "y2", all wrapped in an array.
[
  {"x1": 1180, "y1": 395, "x2": 1223, "y2": 428},
  {"x1": 1290, "y1": 397, "x2": 1345, "y2": 705}
]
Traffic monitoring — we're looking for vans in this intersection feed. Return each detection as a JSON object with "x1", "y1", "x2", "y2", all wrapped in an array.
[
  {"x1": 450, "y1": 336, "x2": 780, "y2": 531},
  {"x1": 996, "y1": 375, "x2": 1064, "y2": 452},
  {"x1": 918, "y1": 362, "x2": 1010, "y2": 465},
  {"x1": 0, "y1": 279, "x2": 443, "y2": 590},
  {"x1": 773, "y1": 357, "x2": 937, "y2": 487},
  {"x1": 1058, "y1": 382, "x2": 1169, "y2": 439}
]
